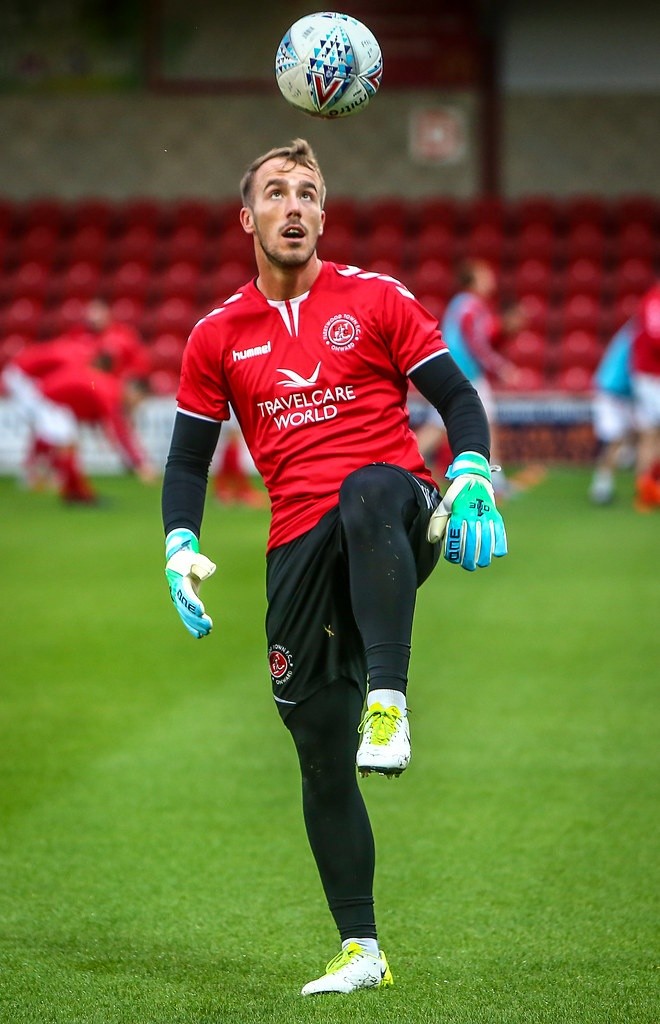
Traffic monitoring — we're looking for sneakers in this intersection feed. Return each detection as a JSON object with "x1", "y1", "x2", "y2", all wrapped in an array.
[
  {"x1": 300, "y1": 942, "x2": 394, "y2": 997},
  {"x1": 356, "y1": 702, "x2": 413, "y2": 780}
]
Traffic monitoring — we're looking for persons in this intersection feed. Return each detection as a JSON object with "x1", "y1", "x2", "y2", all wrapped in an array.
[
  {"x1": 0, "y1": 293, "x2": 152, "y2": 500},
  {"x1": 416, "y1": 265, "x2": 520, "y2": 500},
  {"x1": 215, "y1": 404, "x2": 263, "y2": 506},
  {"x1": 590, "y1": 256, "x2": 660, "y2": 511},
  {"x1": 163, "y1": 138, "x2": 509, "y2": 994}
]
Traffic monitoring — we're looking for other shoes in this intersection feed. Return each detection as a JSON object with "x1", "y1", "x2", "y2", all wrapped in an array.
[
  {"x1": 588, "y1": 466, "x2": 617, "y2": 506},
  {"x1": 489, "y1": 463, "x2": 509, "y2": 498}
]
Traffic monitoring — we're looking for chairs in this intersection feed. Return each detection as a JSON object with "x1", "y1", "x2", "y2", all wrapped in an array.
[{"x1": 0, "y1": 190, "x2": 660, "y2": 462}]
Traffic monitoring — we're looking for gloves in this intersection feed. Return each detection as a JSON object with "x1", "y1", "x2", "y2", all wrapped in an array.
[
  {"x1": 165, "y1": 528, "x2": 217, "y2": 639},
  {"x1": 426, "y1": 451, "x2": 509, "y2": 571}
]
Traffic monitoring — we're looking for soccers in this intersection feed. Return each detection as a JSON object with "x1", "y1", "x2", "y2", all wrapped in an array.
[{"x1": 275, "y1": 11, "x2": 382, "y2": 120}]
